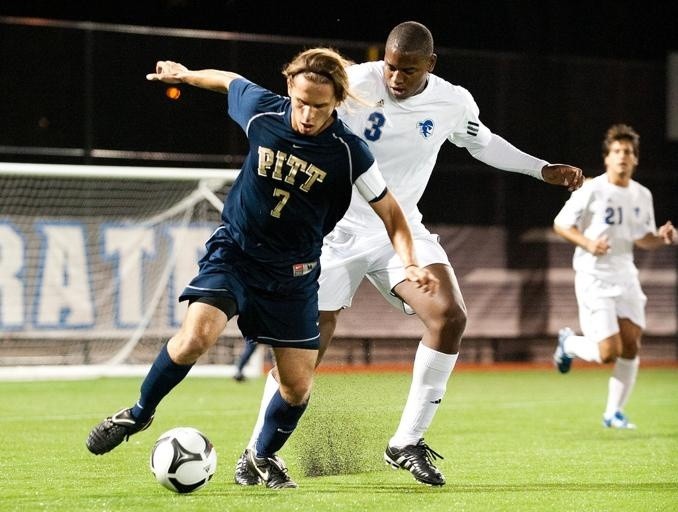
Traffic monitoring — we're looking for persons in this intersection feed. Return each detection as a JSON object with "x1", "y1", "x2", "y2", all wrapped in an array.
[
  {"x1": 552, "y1": 123, "x2": 678, "y2": 429},
  {"x1": 234, "y1": 20, "x2": 585, "y2": 489},
  {"x1": 87, "y1": 46, "x2": 440, "y2": 490}
]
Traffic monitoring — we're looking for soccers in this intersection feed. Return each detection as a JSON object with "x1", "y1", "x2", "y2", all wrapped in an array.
[{"x1": 148, "y1": 427, "x2": 218, "y2": 493}]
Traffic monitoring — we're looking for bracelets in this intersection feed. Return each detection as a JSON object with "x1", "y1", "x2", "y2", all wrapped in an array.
[{"x1": 405, "y1": 264, "x2": 418, "y2": 270}]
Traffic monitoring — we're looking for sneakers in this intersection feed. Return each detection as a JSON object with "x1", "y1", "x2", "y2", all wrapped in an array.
[
  {"x1": 602, "y1": 409, "x2": 637, "y2": 430},
  {"x1": 552, "y1": 326, "x2": 575, "y2": 373},
  {"x1": 383, "y1": 442, "x2": 446, "y2": 486},
  {"x1": 234, "y1": 439, "x2": 298, "y2": 489},
  {"x1": 86, "y1": 406, "x2": 155, "y2": 454},
  {"x1": 233, "y1": 371, "x2": 245, "y2": 381}
]
